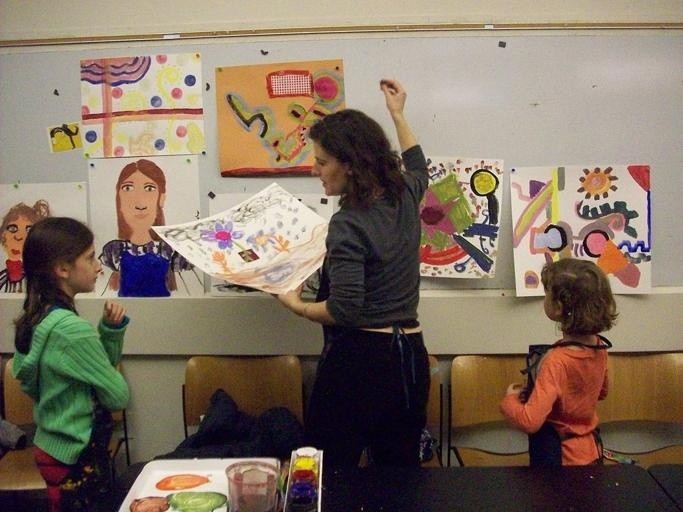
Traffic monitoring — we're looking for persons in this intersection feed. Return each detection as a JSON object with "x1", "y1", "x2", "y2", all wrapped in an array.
[
  {"x1": 270, "y1": 76, "x2": 431, "y2": 463},
  {"x1": 6, "y1": 217, "x2": 132, "y2": 511},
  {"x1": 498, "y1": 258, "x2": 620, "y2": 465}
]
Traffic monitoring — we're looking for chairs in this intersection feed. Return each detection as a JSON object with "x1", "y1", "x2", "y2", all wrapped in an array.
[{"x1": 1, "y1": 357, "x2": 133, "y2": 492}]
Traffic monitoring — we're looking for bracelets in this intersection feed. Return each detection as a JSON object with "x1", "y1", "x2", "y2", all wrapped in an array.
[{"x1": 302, "y1": 301, "x2": 311, "y2": 321}]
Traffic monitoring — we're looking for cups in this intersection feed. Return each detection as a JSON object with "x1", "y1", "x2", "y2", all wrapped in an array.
[{"x1": 225, "y1": 460, "x2": 279, "y2": 512}]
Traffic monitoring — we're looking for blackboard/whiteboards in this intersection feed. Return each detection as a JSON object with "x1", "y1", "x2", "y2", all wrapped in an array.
[{"x1": 0, "y1": 23, "x2": 683, "y2": 301}]
